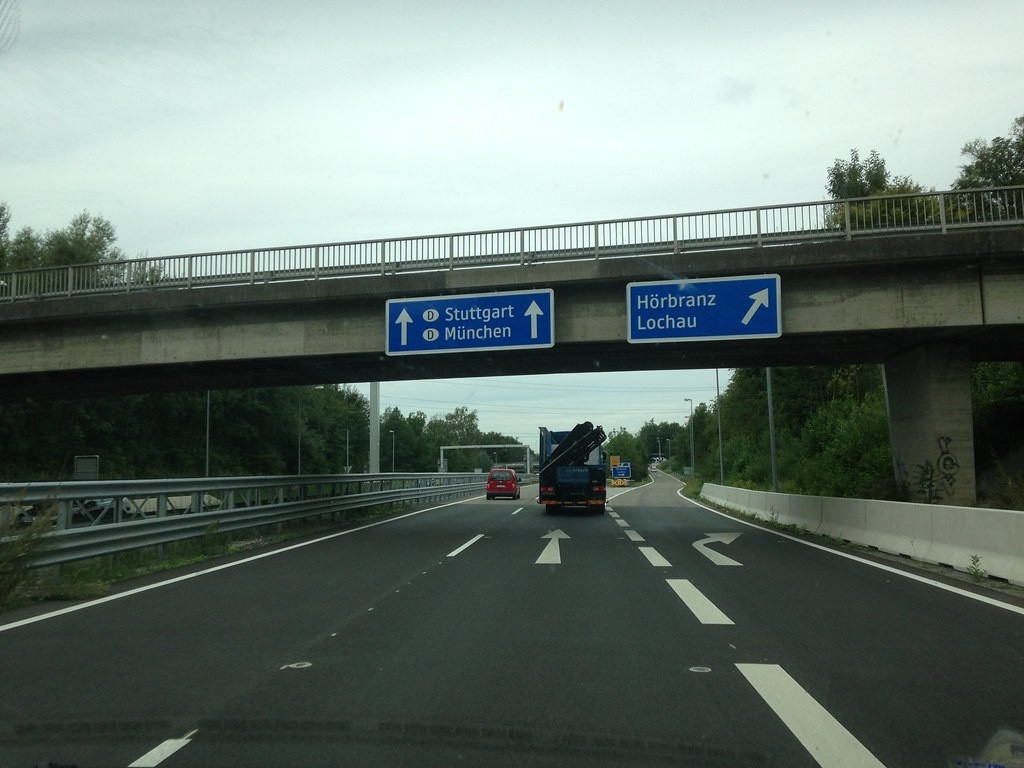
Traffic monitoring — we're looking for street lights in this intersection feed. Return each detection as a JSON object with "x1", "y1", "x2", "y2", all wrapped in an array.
[
  {"x1": 666, "y1": 438, "x2": 671, "y2": 473},
  {"x1": 658, "y1": 440, "x2": 661, "y2": 460},
  {"x1": 494, "y1": 452, "x2": 497, "y2": 467},
  {"x1": 389, "y1": 430, "x2": 395, "y2": 472},
  {"x1": 685, "y1": 416, "x2": 693, "y2": 473},
  {"x1": 684, "y1": 398, "x2": 694, "y2": 481}
]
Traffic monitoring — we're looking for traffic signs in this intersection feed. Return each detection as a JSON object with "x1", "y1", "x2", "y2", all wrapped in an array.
[
  {"x1": 383, "y1": 288, "x2": 553, "y2": 357},
  {"x1": 627, "y1": 274, "x2": 781, "y2": 345}
]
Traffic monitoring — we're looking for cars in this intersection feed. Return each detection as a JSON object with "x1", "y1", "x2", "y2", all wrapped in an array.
[
  {"x1": 652, "y1": 467, "x2": 657, "y2": 473},
  {"x1": 486, "y1": 468, "x2": 521, "y2": 499}
]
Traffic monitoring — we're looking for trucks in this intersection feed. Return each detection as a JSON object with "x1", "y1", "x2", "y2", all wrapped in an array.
[{"x1": 536, "y1": 430, "x2": 607, "y2": 514}]
